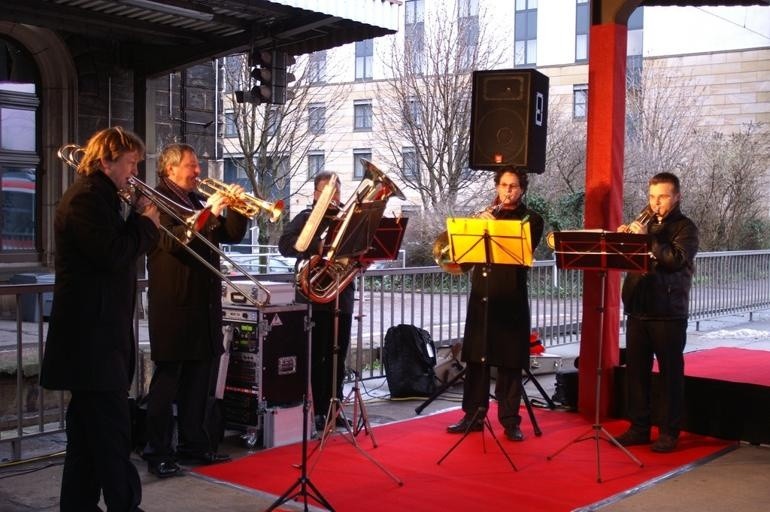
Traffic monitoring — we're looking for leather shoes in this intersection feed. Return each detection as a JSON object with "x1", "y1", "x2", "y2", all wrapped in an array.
[
  {"x1": 447, "y1": 415, "x2": 484, "y2": 432},
  {"x1": 148, "y1": 461, "x2": 179, "y2": 478},
  {"x1": 504, "y1": 428, "x2": 523, "y2": 440},
  {"x1": 315, "y1": 415, "x2": 326, "y2": 430},
  {"x1": 652, "y1": 431, "x2": 679, "y2": 452},
  {"x1": 608, "y1": 430, "x2": 651, "y2": 447},
  {"x1": 336, "y1": 413, "x2": 352, "y2": 426},
  {"x1": 175, "y1": 450, "x2": 231, "y2": 466}
]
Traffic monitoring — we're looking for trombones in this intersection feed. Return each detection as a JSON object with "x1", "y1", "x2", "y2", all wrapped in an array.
[{"x1": 54, "y1": 140, "x2": 273, "y2": 312}]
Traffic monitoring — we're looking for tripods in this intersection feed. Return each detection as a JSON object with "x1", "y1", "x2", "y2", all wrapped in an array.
[
  {"x1": 320, "y1": 277, "x2": 379, "y2": 449},
  {"x1": 436, "y1": 272, "x2": 521, "y2": 473},
  {"x1": 547, "y1": 276, "x2": 645, "y2": 485},
  {"x1": 415, "y1": 348, "x2": 556, "y2": 437},
  {"x1": 291, "y1": 285, "x2": 405, "y2": 488},
  {"x1": 260, "y1": 301, "x2": 338, "y2": 512}
]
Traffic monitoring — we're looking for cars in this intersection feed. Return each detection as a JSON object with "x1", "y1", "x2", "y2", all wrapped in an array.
[{"x1": 241, "y1": 258, "x2": 295, "y2": 273}]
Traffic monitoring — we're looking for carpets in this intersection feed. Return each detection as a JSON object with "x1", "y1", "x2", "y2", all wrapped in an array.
[
  {"x1": 622, "y1": 347, "x2": 770, "y2": 385},
  {"x1": 188, "y1": 399, "x2": 738, "y2": 512}
]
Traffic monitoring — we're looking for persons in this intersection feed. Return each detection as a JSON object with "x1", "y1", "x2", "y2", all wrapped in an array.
[
  {"x1": 445, "y1": 166, "x2": 543, "y2": 442},
  {"x1": 279, "y1": 174, "x2": 359, "y2": 436},
  {"x1": 607, "y1": 174, "x2": 700, "y2": 451},
  {"x1": 41, "y1": 127, "x2": 160, "y2": 512},
  {"x1": 142, "y1": 145, "x2": 248, "y2": 477}
]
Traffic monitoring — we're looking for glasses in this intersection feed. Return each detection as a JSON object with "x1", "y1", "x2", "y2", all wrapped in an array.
[
  {"x1": 315, "y1": 188, "x2": 340, "y2": 195},
  {"x1": 497, "y1": 183, "x2": 520, "y2": 189}
]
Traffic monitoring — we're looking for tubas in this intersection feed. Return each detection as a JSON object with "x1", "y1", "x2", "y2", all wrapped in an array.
[{"x1": 293, "y1": 154, "x2": 408, "y2": 304}]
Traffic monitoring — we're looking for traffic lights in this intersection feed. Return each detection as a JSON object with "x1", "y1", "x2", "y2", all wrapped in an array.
[{"x1": 249, "y1": 47, "x2": 297, "y2": 106}]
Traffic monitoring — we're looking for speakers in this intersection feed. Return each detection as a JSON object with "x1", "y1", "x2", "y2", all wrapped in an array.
[{"x1": 468, "y1": 67, "x2": 550, "y2": 174}]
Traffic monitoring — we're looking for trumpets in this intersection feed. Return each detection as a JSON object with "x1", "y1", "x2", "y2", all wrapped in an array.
[{"x1": 190, "y1": 173, "x2": 289, "y2": 225}]
